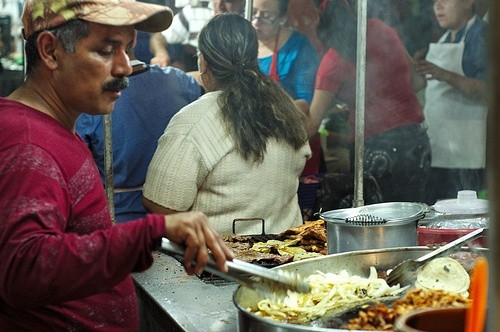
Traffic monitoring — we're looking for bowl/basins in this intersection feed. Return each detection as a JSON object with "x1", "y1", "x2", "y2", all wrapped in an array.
[{"x1": 417, "y1": 228, "x2": 489, "y2": 248}]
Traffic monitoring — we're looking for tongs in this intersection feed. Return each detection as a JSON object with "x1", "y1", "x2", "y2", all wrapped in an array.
[
  {"x1": 153, "y1": 237, "x2": 313, "y2": 301},
  {"x1": 386, "y1": 227, "x2": 489, "y2": 288}
]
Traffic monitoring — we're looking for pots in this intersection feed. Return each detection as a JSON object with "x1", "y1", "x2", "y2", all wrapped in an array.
[
  {"x1": 320, "y1": 202, "x2": 430, "y2": 254},
  {"x1": 232, "y1": 246, "x2": 490, "y2": 332}
]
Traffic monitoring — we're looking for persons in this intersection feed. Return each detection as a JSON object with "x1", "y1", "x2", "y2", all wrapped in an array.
[
  {"x1": 302, "y1": 0, "x2": 432, "y2": 210},
  {"x1": 73, "y1": 0, "x2": 327, "y2": 224},
  {"x1": 416, "y1": 0, "x2": 491, "y2": 201},
  {"x1": 0, "y1": 0, "x2": 233, "y2": 332},
  {"x1": 142, "y1": 13, "x2": 312, "y2": 236}
]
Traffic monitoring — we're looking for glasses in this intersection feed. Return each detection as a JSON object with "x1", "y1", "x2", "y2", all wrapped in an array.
[{"x1": 252, "y1": 12, "x2": 282, "y2": 25}]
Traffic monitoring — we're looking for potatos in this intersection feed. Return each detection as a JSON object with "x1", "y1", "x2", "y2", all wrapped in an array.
[{"x1": 254, "y1": 265, "x2": 413, "y2": 322}]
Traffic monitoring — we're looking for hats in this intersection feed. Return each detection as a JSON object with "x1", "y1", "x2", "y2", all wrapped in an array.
[{"x1": 20, "y1": 0, "x2": 174, "y2": 40}]
